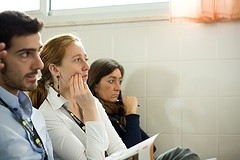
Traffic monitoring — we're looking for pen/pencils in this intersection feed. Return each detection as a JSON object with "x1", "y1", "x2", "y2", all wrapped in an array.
[{"x1": 116, "y1": 100, "x2": 140, "y2": 107}]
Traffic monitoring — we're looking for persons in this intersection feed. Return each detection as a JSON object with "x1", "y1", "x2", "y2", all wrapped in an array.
[
  {"x1": 86, "y1": 58, "x2": 156, "y2": 157},
  {"x1": 29, "y1": 33, "x2": 127, "y2": 160},
  {"x1": 0, "y1": 11, "x2": 201, "y2": 160}
]
